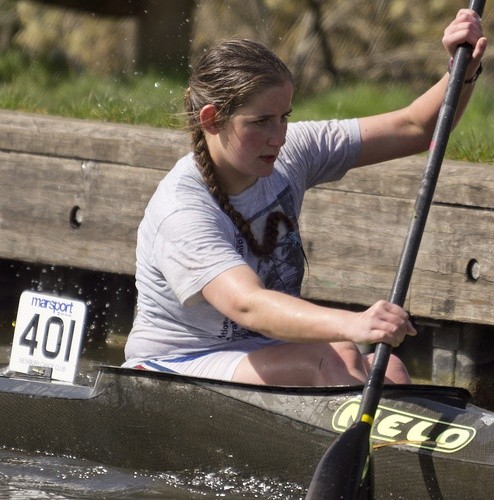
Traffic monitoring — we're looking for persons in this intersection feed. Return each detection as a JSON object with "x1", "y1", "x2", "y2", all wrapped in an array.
[{"x1": 123, "y1": 8, "x2": 488, "y2": 387}]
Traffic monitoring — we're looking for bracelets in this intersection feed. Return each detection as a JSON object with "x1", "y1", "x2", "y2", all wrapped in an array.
[{"x1": 448, "y1": 60, "x2": 482, "y2": 84}]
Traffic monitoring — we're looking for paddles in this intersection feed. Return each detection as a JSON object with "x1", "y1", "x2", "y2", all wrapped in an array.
[{"x1": 306, "y1": 1, "x2": 485, "y2": 499}]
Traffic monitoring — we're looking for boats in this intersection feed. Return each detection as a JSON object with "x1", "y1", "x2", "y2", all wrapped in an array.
[{"x1": 0, "y1": 367, "x2": 494, "y2": 499}]
